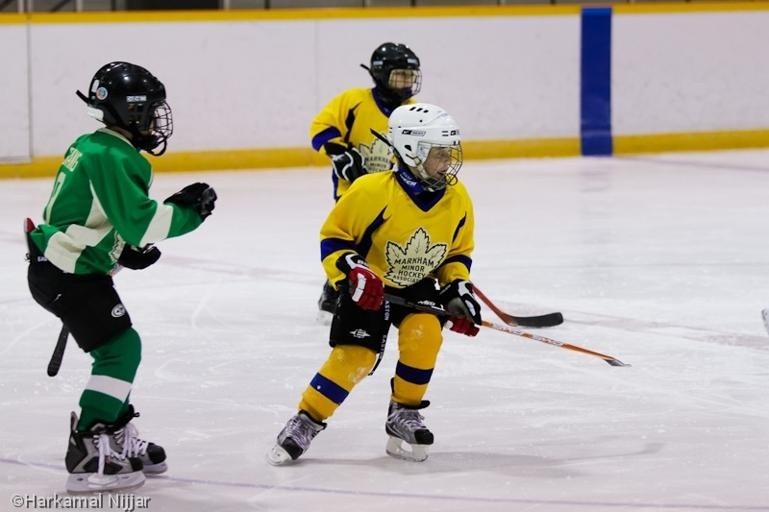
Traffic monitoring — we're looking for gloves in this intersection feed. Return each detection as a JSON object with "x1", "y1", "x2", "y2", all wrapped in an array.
[
  {"x1": 332, "y1": 147, "x2": 368, "y2": 182},
  {"x1": 440, "y1": 279, "x2": 482, "y2": 336},
  {"x1": 348, "y1": 265, "x2": 384, "y2": 311},
  {"x1": 117, "y1": 242, "x2": 160, "y2": 269},
  {"x1": 164, "y1": 183, "x2": 216, "y2": 221}
]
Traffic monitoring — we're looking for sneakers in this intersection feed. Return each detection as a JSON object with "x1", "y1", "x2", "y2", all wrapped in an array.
[
  {"x1": 278, "y1": 411, "x2": 327, "y2": 460},
  {"x1": 385, "y1": 399, "x2": 434, "y2": 444},
  {"x1": 66, "y1": 403, "x2": 166, "y2": 474}
]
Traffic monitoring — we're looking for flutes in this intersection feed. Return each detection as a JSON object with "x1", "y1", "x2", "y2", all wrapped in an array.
[{"x1": 383, "y1": 295, "x2": 629, "y2": 366}]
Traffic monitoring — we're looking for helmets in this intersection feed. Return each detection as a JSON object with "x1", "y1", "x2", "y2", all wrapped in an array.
[
  {"x1": 388, "y1": 103, "x2": 463, "y2": 192},
  {"x1": 77, "y1": 61, "x2": 173, "y2": 156},
  {"x1": 371, "y1": 43, "x2": 421, "y2": 100}
]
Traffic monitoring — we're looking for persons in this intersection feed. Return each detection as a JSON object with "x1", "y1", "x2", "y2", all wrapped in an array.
[
  {"x1": 307, "y1": 43, "x2": 421, "y2": 313},
  {"x1": 25, "y1": 60, "x2": 218, "y2": 475},
  {"x1": 277, "y1": 103, "x2": 481, "y2": 461}
]
